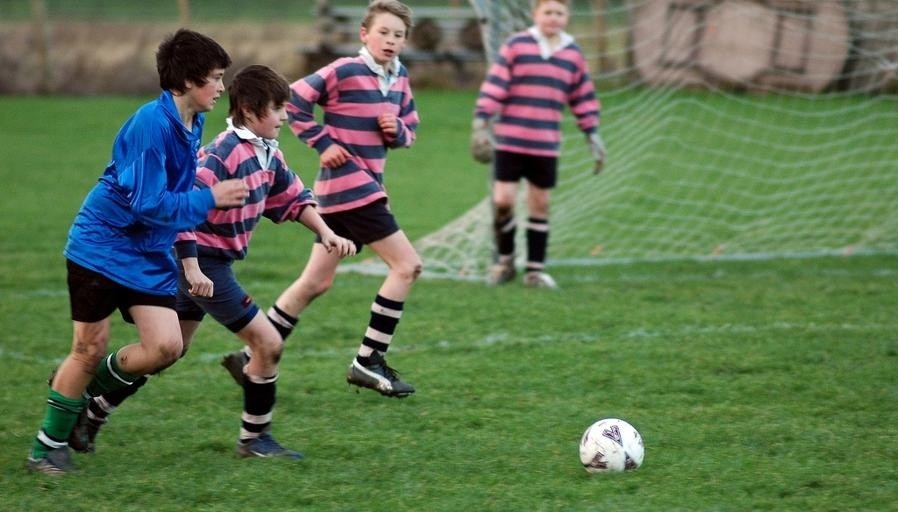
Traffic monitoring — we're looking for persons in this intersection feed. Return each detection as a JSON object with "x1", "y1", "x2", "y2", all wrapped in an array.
[
  {"x1": 304, "y1": 0, "x2": 485, "y2": 86},
  {"x1": 48, "y1": 62, "x2": 359, "y2": 462},
  {"x1": 28, "y1": 27, "x2": 253, "y2": 481},
  {"x1": 221, "y1": 0, "x2": 425, "y2": 399},
  {"x1": 468, "y1": 0, "x2": 606, "y2": 292}
]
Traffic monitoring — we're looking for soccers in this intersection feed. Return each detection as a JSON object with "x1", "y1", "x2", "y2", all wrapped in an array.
[{"x1": 579, "y1": 418, "x2": 644, "y2": 473}]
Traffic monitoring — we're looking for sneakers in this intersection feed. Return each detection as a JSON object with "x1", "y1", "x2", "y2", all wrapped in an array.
[
  {"x1": 220, "y1": 351, "x2": 250, "y2": 389},
  {"x1": 344, "y1": 348, "x2": 417, "y2": 399},
  {"x1": 25, "y1": 446, "x2": 76, "y2": 477},
  {"x1": 482, "y1": 263, "x2": 517, "y2": 287},
  {"x1": 70, "y1": 407, "x2": 108, "y2": 456},
  {"x1": 233, "y1": 431, "x2": 304, "y2": 460},
  {"x1": 523, "y1": 267, "x2": 557, "y2": 290}
]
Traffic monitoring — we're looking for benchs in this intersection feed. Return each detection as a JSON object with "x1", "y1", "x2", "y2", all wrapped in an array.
[{"x1": 321, "y1": 3, "x2": 486, "y2": 85}]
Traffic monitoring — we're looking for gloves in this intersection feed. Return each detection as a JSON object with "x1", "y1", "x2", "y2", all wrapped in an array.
[
  {"x1": 586, "y1": 133, "x2": 608, "y2": 174},
  {"x1": 470, "y1": 130, "x2": 497, "y2": 165}
]
